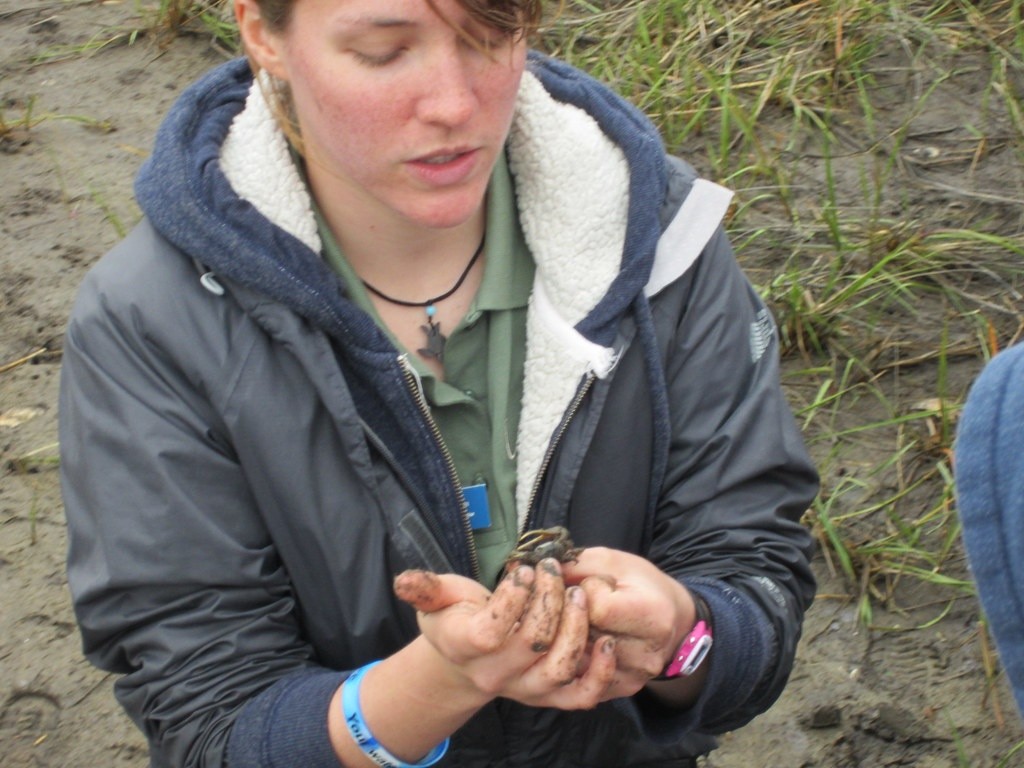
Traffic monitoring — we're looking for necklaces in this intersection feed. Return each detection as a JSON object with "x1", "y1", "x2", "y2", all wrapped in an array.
[{"x1": 359, "y1": 236, "x2": 485, "y2": 361}]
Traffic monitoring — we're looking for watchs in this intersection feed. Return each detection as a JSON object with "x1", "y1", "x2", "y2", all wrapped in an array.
[{"x1": 653, "y1": 591, "x2": 713, "y2": 681}]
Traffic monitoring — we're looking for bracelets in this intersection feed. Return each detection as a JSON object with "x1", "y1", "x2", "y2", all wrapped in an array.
[{"x1": 342, "y1": 660, "x2": 448, "y2": 768}]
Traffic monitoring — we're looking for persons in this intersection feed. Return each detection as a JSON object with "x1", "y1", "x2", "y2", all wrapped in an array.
[{"x1": 61, "y1": 0, "x2": 820, "y2": 768}]
